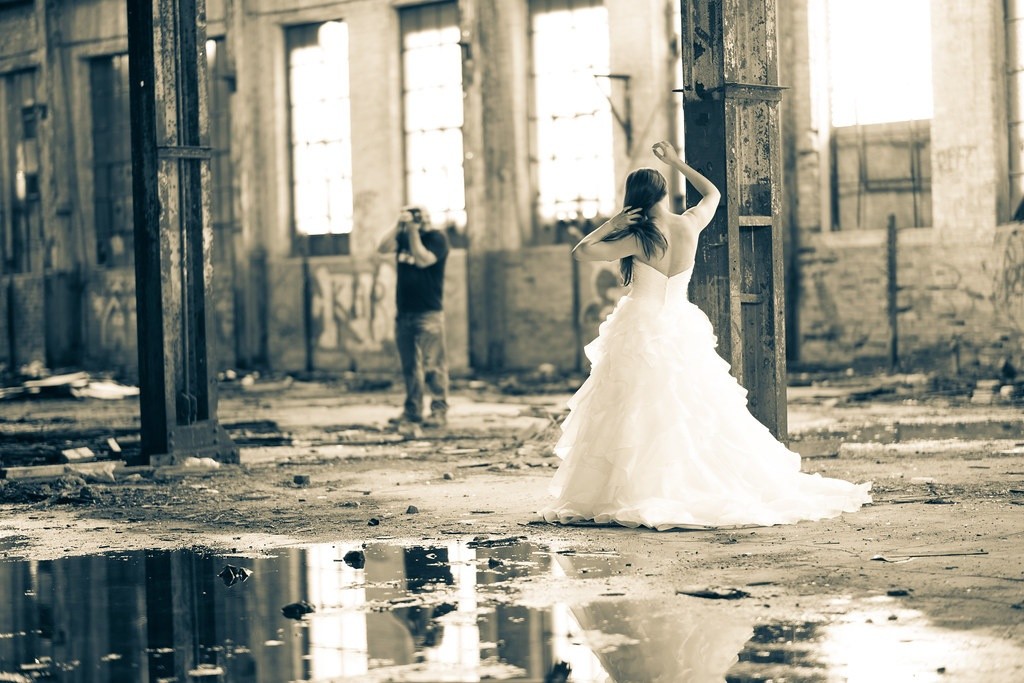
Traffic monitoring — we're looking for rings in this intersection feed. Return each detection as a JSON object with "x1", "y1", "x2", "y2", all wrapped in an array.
[{"x1": 628, "y1": 211, "x2": 631, "y2": 215}]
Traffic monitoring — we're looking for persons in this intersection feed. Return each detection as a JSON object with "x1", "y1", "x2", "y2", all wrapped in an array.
[
  {"x1": 569, "y1": 137, "x2": 721, "y2": 523},
  {"x1": 369, "y1": 206, "x2": 449, "y2": 425}
]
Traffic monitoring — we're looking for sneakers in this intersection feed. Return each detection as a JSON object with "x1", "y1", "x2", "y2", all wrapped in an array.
[
  {"x1": 422, "y1": 412, "x2": 447, "y2": 426},
  {"x1": 388, "y1": 412, "x2": 425, "y2": 424}
]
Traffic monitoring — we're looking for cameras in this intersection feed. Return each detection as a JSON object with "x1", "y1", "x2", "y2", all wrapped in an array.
[{"x1": 408, "y1": 209, "x2": 422, "y2": 224}]
